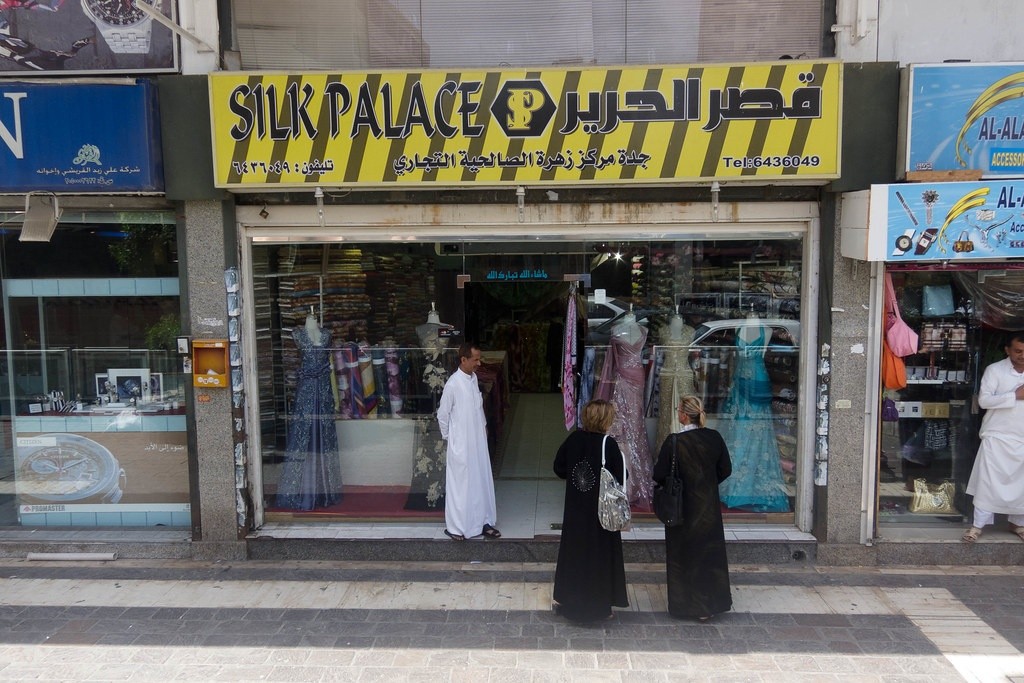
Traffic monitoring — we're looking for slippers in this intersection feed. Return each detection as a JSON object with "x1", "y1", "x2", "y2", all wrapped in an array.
[
  {"x1": 1008, "y1": 526, "x2": 1024, "y2": 541},
  {"x1": 445, "y1": 528, "x2": 465, "y2": 541},
  {"x1": 963, "y1": 526, "x2": 983, "y2": 542},
  {"x1": 481, "y1": 524, "x2": 501, "y2": 540}
]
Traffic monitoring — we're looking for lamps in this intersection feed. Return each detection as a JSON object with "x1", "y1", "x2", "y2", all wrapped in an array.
[
  {"x1": 710, "y1": 181, "x2": 720, "y2": 222},
  {"x1": 18, "y1": 190, "x2": 63, "y2": 242},
  {"x1": 315, "y1": 187, "x2": 324, "y2": 223},
  {"x1": 516, "y1": 185, "x2": 526, "y2": 222}
]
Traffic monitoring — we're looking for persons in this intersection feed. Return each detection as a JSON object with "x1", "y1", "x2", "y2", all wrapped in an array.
[
  {"x1": 437, "y1": 343, "x2": 501, "y2": 540},
  {"x1": 554, "y1": 398, "x2": 629, "y2": 621},
  {"x1": 546, "y1": 312, "x2": 564, "y2": 393},
  {"x1": 652, "y1": 395, "x2": 733, "y2": 621},
  {"x1": 594, "y1": 311, "x2": 790, "y2": 513},
  {"x1": 403, "y1": 311, "x2": 455, "y2": 513},
  {"x1": 273, "y1": 314, "x2": 344, "y2": 511},
  {"x1": 963, "y1": 332, "x2": 1024, "y2": 542}
]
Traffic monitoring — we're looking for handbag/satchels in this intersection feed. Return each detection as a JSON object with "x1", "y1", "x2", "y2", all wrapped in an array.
[
  {"x1": 908, "y1": 478, "x2": 958, "y2": 514},
  {"x1": 903, "y1": 445, "x2": 931, "y2": 467},
  {"x1": 922, "y1": 320, "x2": 967, "y2": 353},
  {"x1": 597, "y1": 468, "x2": 630, "y2": 531},
  {"x1": 882, "y1": 398, "x2": 899, "y2": 422},
  {"x1": 885, "y1": 314, "x2": 918, "y2": 357},
  {"x1": 925, "y1": 420, "x2": 948, "y2": 450},
  {"x1": 883, "y1": 339, "x2": 907, "y2": 390},
  {"x1": 922, "y1": 285, "x2": 955, "y2": 317},
  {"x1": 653, "y1": 476, "x2": 685, "y2": 528}
]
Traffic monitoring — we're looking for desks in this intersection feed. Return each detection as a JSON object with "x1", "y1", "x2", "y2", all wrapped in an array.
[{"x1": 456, "y1": 350, "x2": 512, "y2": 461}]
